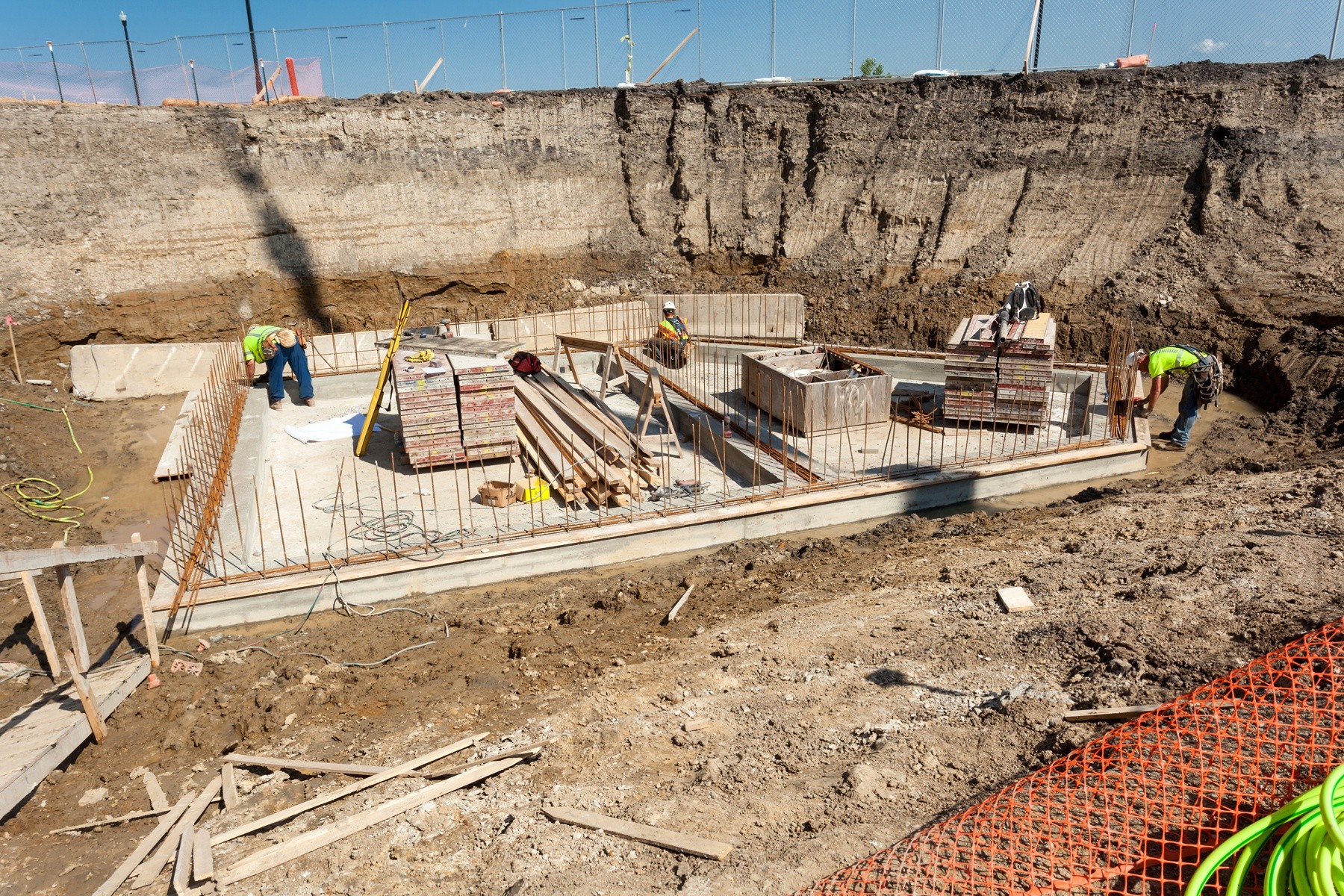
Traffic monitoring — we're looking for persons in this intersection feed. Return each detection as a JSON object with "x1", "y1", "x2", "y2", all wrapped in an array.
[
  {"x1": 243, "y1": 323, "x2": 316, "y2": 410},
  {"x1": 1126, "y1": 343, "x2": 1223, "y2": 452},
  {"x1": 648, "y1": 300, "x2": 696, "y2": 365}
]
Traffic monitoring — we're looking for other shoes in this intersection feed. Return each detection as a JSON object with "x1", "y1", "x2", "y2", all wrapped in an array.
[
  {"x1": 305, "y1": 398, "x2": 314, "y2": 406},
  {"x1": 271, "y1": 399, "x2": 282, "y2": 410},
  {"x1": 1159, "y1": 432, "x2": 1174, "y2": 440},
  {"x1": 1157, "y1": 442, "x2": 1186, "y2": 452}
]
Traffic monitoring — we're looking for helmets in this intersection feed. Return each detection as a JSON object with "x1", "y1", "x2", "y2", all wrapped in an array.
[
  {"x1": 1126, "y1": 348, "x2": 1145, "y2": 368},
  {"x1": 247, "y1": 324, "x2": 261, "y2": 334},
  {"x1": 663, "y1": 302, "x2": 676, "y2": 310}
]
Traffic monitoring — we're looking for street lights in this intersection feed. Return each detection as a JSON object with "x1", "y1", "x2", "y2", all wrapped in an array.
[{"x1": 119, "y1": 11, "x2": 142, "y2": 105}]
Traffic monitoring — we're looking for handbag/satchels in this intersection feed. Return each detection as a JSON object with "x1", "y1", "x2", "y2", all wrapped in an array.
[
  {"x1": 262, "y1": 329, "x2": 307, "y2": 360},
  {"x1": 671, "y1": 340, "x2": 697, "y2": 358},
  {"x1": 507, "y1": 351, "x2": 542, "y2": 376}
]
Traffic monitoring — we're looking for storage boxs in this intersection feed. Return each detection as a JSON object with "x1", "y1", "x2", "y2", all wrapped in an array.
[
  {"x1": 515, "y1": 476, "x2": 550, "y2": 504},
  {"x1": 477, "y1": 480, "x2": 517, "y2": 507}
]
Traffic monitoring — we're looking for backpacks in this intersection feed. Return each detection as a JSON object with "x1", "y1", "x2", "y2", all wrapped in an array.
[{"x1": 1014, "y1": 280, "x2": 1040, "y2": 321}]
